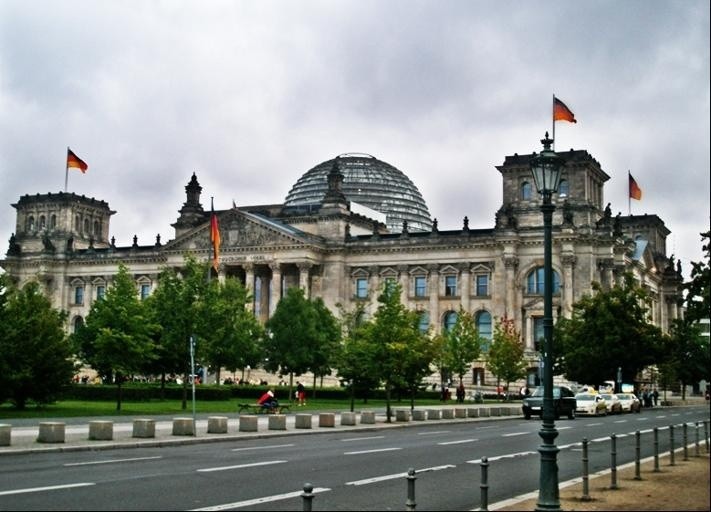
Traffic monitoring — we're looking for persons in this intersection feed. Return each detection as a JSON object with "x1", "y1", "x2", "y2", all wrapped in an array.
[
  {"x1": 71, "y1": 370, "x2": 307, "y2": 414},
  {"x1": 430, "y1": 382, "x2": 660, "y2": 408}
]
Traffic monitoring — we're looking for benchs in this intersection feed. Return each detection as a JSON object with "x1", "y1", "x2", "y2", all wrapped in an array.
[{"x1": 238, "y1": 403, "x2": 292, "y2": 415}]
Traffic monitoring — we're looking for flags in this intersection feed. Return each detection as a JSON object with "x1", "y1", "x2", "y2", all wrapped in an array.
[
  {"x1": 67, "y1": 149, "x2": 88, "y2": 174},
  {"x1": 211, "y1": 212, "x2": 221, "y2": 273},
  {"x1": 629, "y1": 173, "x2": 642, "y2": 201},
  {"x1": 554, "y1": 97, "x2": 577, "y2": 124}
]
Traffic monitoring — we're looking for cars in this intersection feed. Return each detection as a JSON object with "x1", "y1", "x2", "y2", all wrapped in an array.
[{"x1": 575, "y1": 393, "x2": 640, "y2": 416}]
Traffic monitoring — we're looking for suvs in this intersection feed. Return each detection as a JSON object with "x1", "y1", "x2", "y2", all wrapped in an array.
[{"x1": 522, "y1": 386, "x2": 576, "y2": 420}]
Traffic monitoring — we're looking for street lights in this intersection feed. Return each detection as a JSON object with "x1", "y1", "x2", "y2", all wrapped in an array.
[{"x1": 531, "y1": 131, "x2": 564, "y2": 512}]
